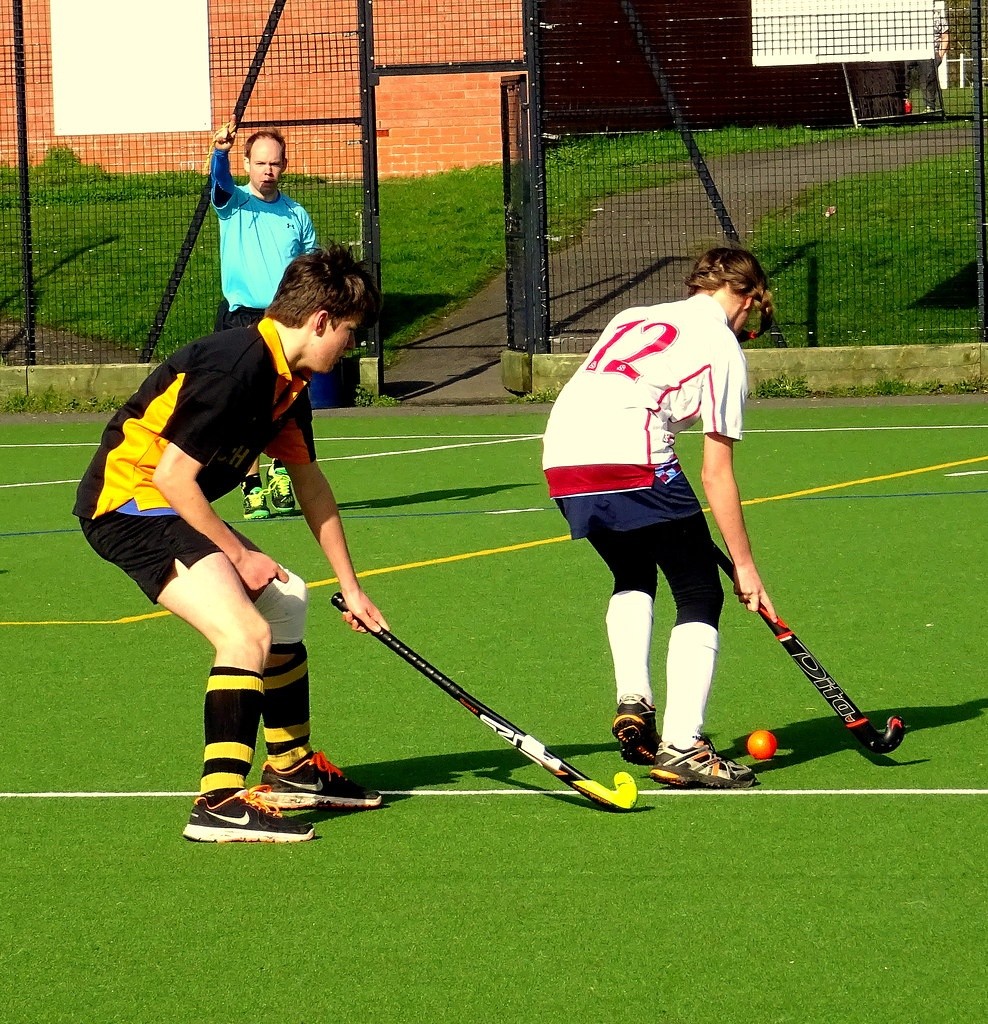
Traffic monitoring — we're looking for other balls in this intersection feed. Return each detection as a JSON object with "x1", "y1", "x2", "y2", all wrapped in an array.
[{"x1": 746, "y1": 729, "x2": 778, "y2": 761}]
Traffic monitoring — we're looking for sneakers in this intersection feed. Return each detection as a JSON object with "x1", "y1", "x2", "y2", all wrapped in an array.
[
  {"x1": 240, "y1": 482, "x2": 271, "y2": 520},
  {"x1": 183, "y1": 784, "x2": 315, "y2": 843},
  {"x1": 650, "y1": 733, "x2": 755, "y2": 789},
  {"x1": 611, "y1": 693, "x2": 662, "y2": 765},
  {"x1": 260, "y1": 751, "x2": 382, "y2": 808},
  {"x1": 266, "y1": 459, "x2": 295, "y2": 513}
]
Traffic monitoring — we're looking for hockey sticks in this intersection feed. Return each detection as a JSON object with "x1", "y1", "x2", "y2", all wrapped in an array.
[
  {"x1": 709, "y1": 538, "x2": 907, "y2": 754},
  {"x1": 330, "y1": 592, "x2": 639, "y2": 813}
]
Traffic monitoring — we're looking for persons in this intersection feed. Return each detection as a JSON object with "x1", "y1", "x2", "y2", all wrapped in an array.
[
  {"x1": 72, "y1": 242, "x2": 393, "y2": 841},
  {"x1": 209, "y1": 114, "x2": 318, "y2": 520},
  {"x1": 904, "y1": 7, "x2": 950, "y2": 113},
  {"x1": 542, "y1": 248, "x2": 777, "y2": 789}
]
